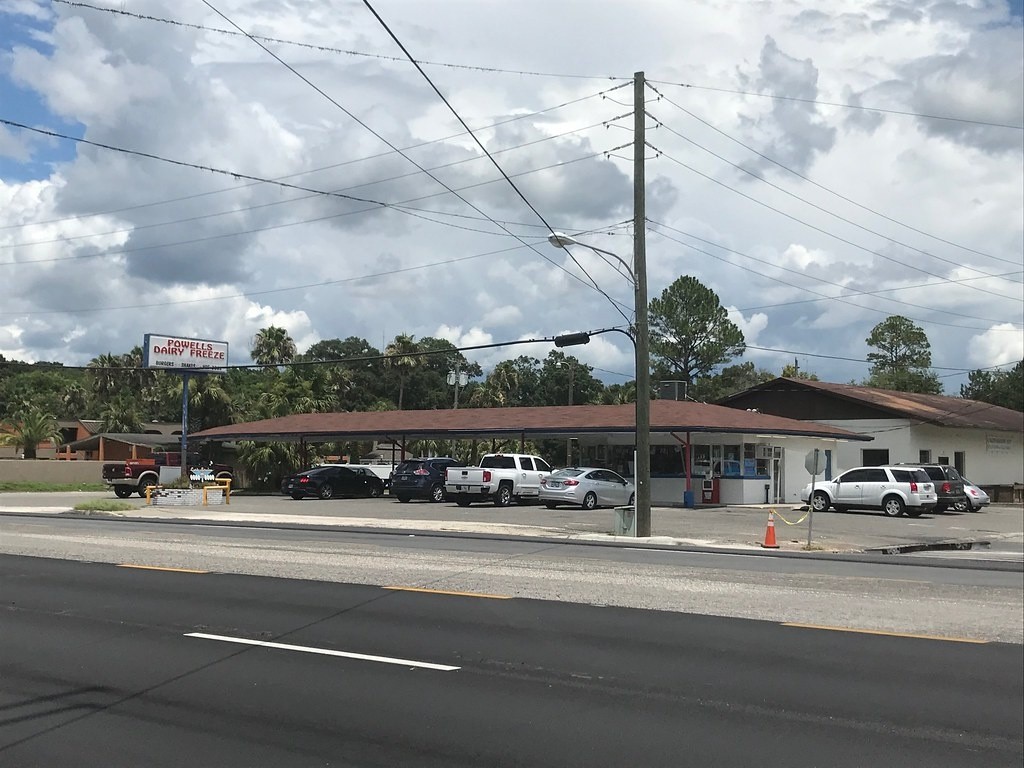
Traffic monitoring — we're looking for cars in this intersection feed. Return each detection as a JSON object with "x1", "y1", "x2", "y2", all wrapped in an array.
[
  {"x1": 952, "y1": 475, "x2": 990, "y2": 513},
  {"x1": 280, "y1": 465, "x2": 385, "y2": 500},
  {"x1": 388, "y1": 456, "x2": 467, "y2": 503},
  {"x1": 537, "y1": 466, "x2": 635, "y2": 510}
]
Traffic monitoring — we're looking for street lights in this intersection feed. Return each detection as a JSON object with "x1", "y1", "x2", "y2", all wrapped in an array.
[
  {"x1": 547, "y1": 232, "x2": 651, "y2": 539},
  {"x1": 760, "y1": 509, "x2": 780, "y2": 548}
]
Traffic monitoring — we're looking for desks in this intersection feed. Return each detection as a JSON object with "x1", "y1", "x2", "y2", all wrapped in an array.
[{"x1": 995, "y1": 485, "x2": 1024, "y2": 503}]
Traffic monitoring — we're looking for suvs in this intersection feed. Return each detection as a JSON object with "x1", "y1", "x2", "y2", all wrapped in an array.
[
  {"x1": 800, "y1": 465, "x2": 938, "y2": 517},
  {"x1": 895, "y1": 462, "x2": 964, "y2": 514}
]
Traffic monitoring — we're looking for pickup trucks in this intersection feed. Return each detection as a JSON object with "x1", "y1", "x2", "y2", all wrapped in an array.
[
  {"x1": 442, "y1": 453, "x2": 559, "y2": 507},
  {"x1": 101, "y1": 451, "x2": 233, "y2": 499}
]
